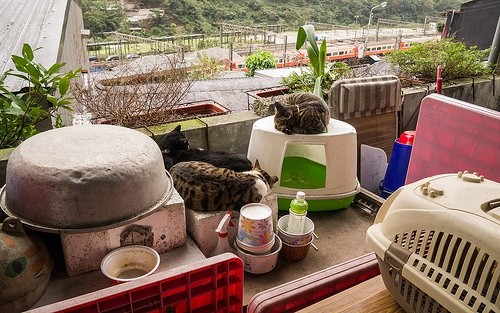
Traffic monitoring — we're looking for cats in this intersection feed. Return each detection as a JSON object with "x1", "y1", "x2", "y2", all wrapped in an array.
[
  {"x1": 272, "y1": 93, "x2": 331, "y2": 135},
  {"x1": 158, "y1": 124, "x2": 279, "y2": 212}
]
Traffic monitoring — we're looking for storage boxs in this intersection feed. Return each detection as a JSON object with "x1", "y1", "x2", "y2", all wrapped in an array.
[
  {"x1": 365, "y1": 169, "x2": 500, "y2": 313},
  {"x1": 59, "y1": 187, "x2": 187, "y2": 271},
  {"x1": 186, "y1": 192, "x2": 278, "y2": 258},
  {"x1": 246, "y1": 116, "x2": 361, "y2": 213}
]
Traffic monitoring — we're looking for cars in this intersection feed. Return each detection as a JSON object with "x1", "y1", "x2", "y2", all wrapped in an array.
[{"x1": 88, "y1": 54, "x2": 139, "y2": 61}]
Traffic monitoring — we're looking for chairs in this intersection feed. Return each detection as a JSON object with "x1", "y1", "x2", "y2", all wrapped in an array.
[{"x1": 327, "y1": 74, "x2": 405, "y2": 164}]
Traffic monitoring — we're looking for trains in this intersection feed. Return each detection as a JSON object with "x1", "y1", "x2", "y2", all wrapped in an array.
[{"x1": 226, "y1": 42, "x2": 411, "y2": 70}]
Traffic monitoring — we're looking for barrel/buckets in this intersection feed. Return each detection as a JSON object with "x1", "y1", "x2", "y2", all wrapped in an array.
[{"x1": 379, "y1": 138, "x2": 412, "y2": 193}]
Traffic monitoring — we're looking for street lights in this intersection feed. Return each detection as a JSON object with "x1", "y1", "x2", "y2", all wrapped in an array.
[{"x1": 365, "y1": 2, "x2": 388, "y2": 42}]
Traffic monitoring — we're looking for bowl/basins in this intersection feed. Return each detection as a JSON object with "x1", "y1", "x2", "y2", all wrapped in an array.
[
  {"x1": 101, "y1": 245, "x2": 160, "y2": 285},
  {"x1": 0, "y1": 124, "x2": 174, "y2": 233}
]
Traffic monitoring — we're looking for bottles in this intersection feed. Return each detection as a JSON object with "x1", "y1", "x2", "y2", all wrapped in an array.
[{"x1": 286, "y1": 191, "x2": 308, "y2": 234}]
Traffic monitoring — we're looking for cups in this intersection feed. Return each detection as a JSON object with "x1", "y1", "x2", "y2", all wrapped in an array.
[
  {"x1": 234, "y1": 202, "x2": 282, "y2": 275},
  {"x1": 278, "y1": 215, "x2": 314, "y2": 261}
]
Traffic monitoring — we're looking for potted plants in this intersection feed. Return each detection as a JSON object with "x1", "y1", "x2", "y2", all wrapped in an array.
[{"x1": 69, "y1": 23, "x2": 349, "y2": 130}]
